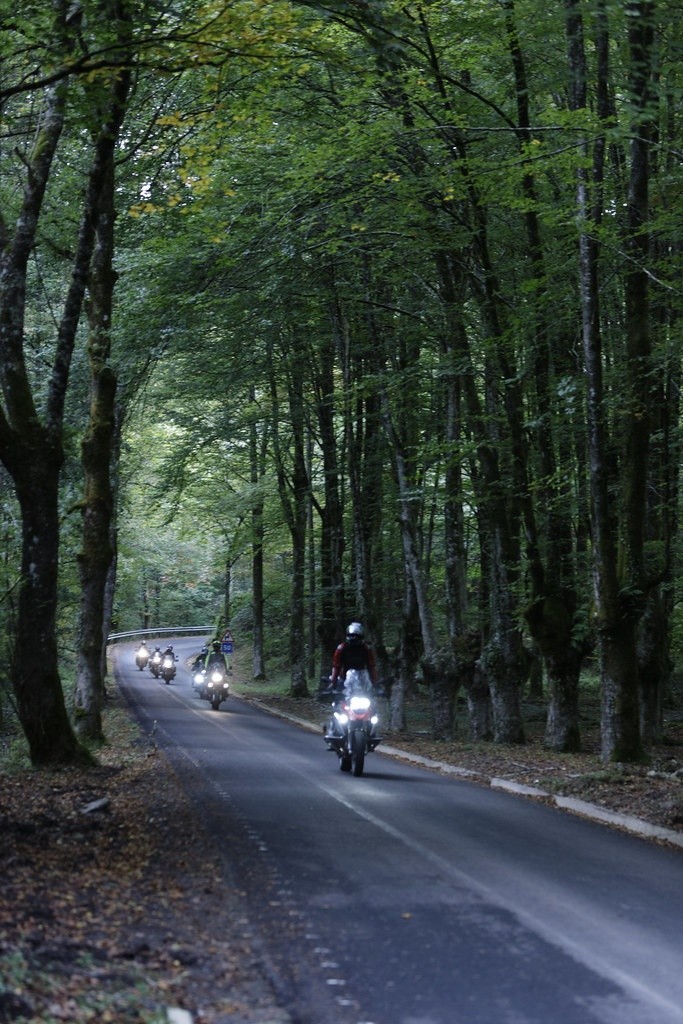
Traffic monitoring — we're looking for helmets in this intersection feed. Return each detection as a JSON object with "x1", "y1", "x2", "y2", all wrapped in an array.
[
  {"x1": 155, "y1": 645, "x2": 160, "y2": 650},
  {"x1": 346, "y1": 622, "x2": 364, "y2": 638},
  {"x1": 213, "y1": 640, "x2": 221, "y2": 648},
  {"x1": 167, "y1": 645, "x2": 172, "y2": 651},
  {"x1": 201, "y1": 647, "x2": 208, "y2": 655},
  {"x1": 141, "y1": 640, "x2": 146, "y2": 644}
]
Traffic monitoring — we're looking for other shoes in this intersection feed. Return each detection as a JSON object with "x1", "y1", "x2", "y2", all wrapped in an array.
[
  {"x1": 368, "y1": 739, "x2": 380, "y2": 751},
  {"x1": 327, "y1": 742, "x2": 334, "y2": 751}
]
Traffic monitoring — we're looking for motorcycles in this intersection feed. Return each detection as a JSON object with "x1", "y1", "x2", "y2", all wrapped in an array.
[
  {"x1": 160, "y1": 654, "x2": 179, "y2": 684},
  {"x1": 320, "y1": 667, "x2": 390, "y2": 777},
  {"x1": 191, "y1": 659, "x2": 211, "y2": 700},
  {"x1": 148, "y1": 651, "x2": 164, "y2": 679},
  {"x1": 202, "y1": 658, "x2": 233, "y2": 710},
  {"x1": 135, "y1": 645, "x2": 151, "y2": 671}
]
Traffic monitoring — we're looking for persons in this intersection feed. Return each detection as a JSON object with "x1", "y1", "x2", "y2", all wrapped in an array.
[
  {"x1": 136, "y1": 640, "x2": 232, "y2": 697},
  {"x1": 327, "y1": 623, "x2": 378, "y2": 750}
]
